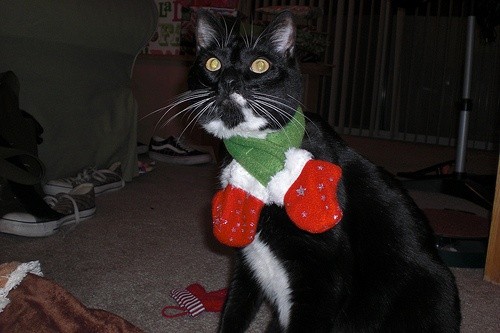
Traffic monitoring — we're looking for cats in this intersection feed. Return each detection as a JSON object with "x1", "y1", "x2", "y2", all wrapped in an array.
[{"x1": 139, "y1": 9, "x2": 463, "y2": 333}]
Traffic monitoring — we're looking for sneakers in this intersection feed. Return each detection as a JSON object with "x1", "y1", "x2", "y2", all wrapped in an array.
[
  {"x1": 42, "y1": 166, "x2": 125, "y2": 198},
  {"x1": 0, "y1": 182, "x2": 96, "y2": 237},
  {"x1": 147, "y1": 135, "x2": 211, "y2": 165},
  {"x1": 106, "y1": 154, "x2": 157, "y2": 182}
]
